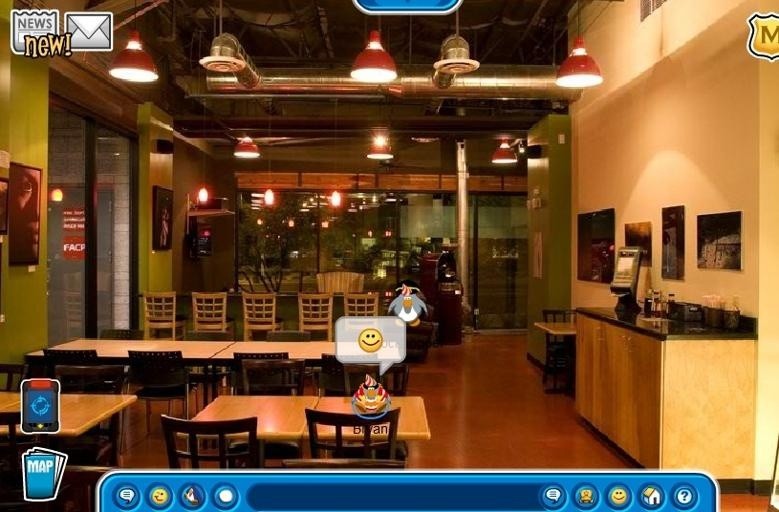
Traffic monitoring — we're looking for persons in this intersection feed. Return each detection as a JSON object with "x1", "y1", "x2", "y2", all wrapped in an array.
[{"x1": 10, "y1": 165, "x2": 40, "y2": 260}]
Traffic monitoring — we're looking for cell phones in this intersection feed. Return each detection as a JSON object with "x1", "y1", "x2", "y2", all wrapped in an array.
[{"x1": 21, "y1": 378, "x2": 61, "y2": 432}]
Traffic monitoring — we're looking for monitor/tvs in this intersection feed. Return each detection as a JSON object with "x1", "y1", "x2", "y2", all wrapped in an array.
[{"x1": 609, "y1": 246, "x2": 642, "y2": 318}]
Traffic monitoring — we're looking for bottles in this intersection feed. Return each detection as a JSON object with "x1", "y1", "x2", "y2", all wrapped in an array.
[
  {"x1": 704, "y1": 295, "x2": 740, "y2": 333},
  {"x1": 644, "y1": 290, "x2": 675, "y2": 318}
]
